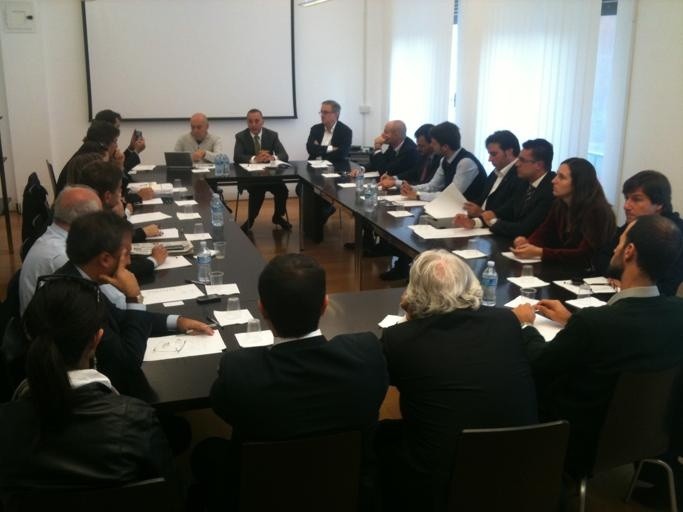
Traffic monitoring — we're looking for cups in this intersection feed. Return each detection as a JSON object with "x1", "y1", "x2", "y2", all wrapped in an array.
[
  {"x1": 214, "y1": 242, "x2": 225, "y2": 257},
  {"x1": 246, "y1": 319, "x2": 260, "y2": 332},
  {"x1": 172, "y1": 177, "x2": 205, "y2": 236},
  {"x1": 211, "y1": 271, "x2": 224, "y2": 285},
  {"x1": 577, "y1": 286, "x2": 592, "y2": 305},
  {"x1": 521, "y1": 287, "x2": 536, "y2": 300},
  {"x1": 226, "y1": 298, "x2": 240, "y2": 311},
  {"x1": 395, "y1": 199, "x2": 536, "y2": 280}
]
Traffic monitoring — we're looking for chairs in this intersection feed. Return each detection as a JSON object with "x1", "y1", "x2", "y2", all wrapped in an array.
[
  {"x1": 238, "y1": 430, "x2": 362, "y2": 512},
  {"x1": 20, "y1": 174, "x2": 49, "y2": 241},
  {"x1": 45, "y1": 159, "x2": 58, "y2": 201},
  {"x1": 579, "y1": 364, "x2": 682, "y2": 512},
  {"x1": 0, "y1": 267, "x2": 23, "y2": 346},
  {"x1": 6, "y1": 477, "x2": 184, "y2": 512},
  {"x1": 448, "y1": 420, "x2": 570, "y2": 512}
]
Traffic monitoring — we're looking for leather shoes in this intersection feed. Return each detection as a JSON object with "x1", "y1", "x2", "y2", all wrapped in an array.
[{"x1": 238, "y1": 216, "x2": 411, "y2": 281}]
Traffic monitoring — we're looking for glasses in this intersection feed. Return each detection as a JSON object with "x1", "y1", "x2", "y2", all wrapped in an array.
[
  {"x1": 35, "y1": 273, "x2": 100, "y2": 312},
  {"x1": 518, "y1": 155, "x2": 536, "y2": 164},
  {"x1": 318, "y1": 110, "x2": 333, "y2": 115}
]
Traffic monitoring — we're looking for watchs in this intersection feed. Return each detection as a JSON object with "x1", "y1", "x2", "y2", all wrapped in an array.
[{"x1": 519, "y1": 321, "x2": 533, "y2": 329}]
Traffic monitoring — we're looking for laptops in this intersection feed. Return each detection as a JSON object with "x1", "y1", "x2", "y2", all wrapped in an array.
[{"x1": 164, "y1": 151, "x2": 194, "y2": 169}]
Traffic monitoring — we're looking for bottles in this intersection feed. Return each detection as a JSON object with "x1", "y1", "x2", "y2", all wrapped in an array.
[
  {"x1": 198, "y1": 241, "x2": 211, "y2": 281},
  {"x1": 483, "y1": 260, "x2": 497, "y2": 307},
  {"x1": 211, "y1": 153, "x2": 379, "y2": 228}
]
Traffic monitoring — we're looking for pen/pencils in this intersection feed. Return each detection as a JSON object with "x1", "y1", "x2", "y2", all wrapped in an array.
[
  {"x1": 184, "y1": 279, "x2": 205, "y2": 285},
  {"x1": 208, "y1": 316, "x2": 224, "y2": 330},
  {"x1": 186, "y1": 324, "x2": 217, "y2": 334}
]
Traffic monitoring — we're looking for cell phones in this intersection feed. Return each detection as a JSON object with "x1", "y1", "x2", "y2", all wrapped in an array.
[
  {"x1": 164, "y1": 244, "x2": 184, "y2": 250},
  {"x1": 196, "y1": 293, "x2": 222, "y2": 303},
  {"x1": 181, "y1": 194, "x2": 193, "y2": 198},
  {"x1": 134, "y1": 130, "x2": 142, "y2": 138}
]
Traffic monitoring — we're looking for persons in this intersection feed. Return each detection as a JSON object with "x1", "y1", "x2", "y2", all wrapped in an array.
[
  {"x1": 379, "y1": 246, "x2": 540, "y2": 511},
  {"x1": 511, "y1": 213, "x2": 682, "y2": 512},
  {"x1": 171, "y1": 112, "x2": 223, "y2": 164},
  {"x1": 344, "y1": 118, "x2": 683, "y2": 294},
  {"x1": 234, "y1": 109, "x2": 292, "y2": 233},
  {"x1": 295, "y1": 100, "x2": 351, "y2": 246},
  {"x1": 5, "y1": 109, "x2": 214, "y2": 510},
  {"x1": 205, "y1": 252, "x2": 389, "y2": 512}
]
